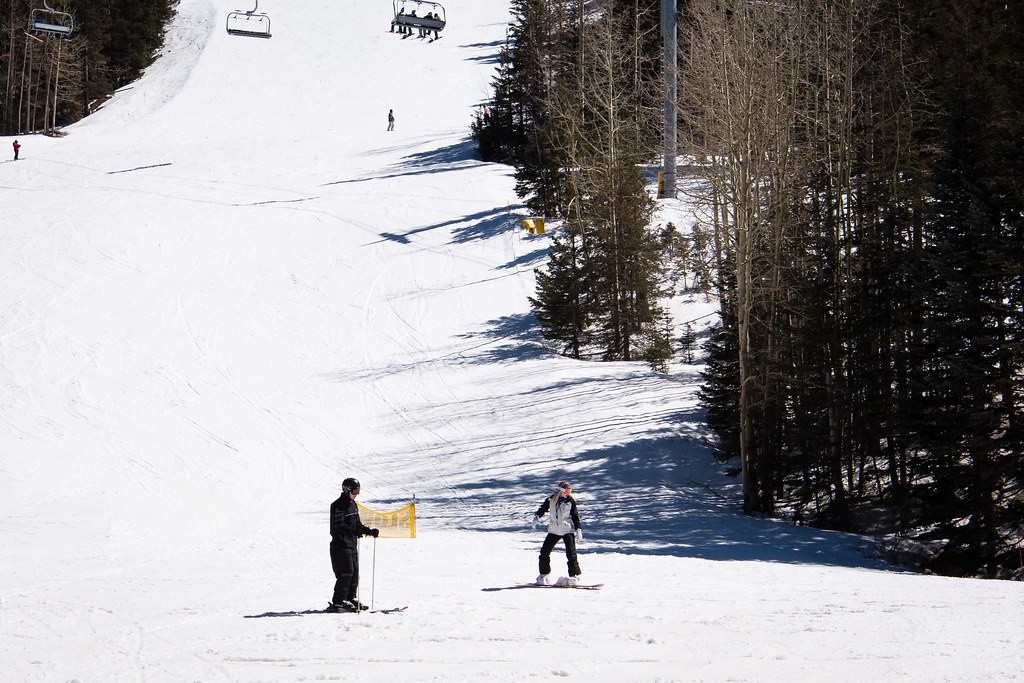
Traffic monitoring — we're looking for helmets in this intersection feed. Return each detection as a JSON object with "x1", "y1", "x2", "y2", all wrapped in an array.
[{"x1": 342, "y1": 478, "x2": 360, "y2": 492}]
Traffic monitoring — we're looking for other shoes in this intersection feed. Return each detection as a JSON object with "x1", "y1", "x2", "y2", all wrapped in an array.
[
  {"x1": 348, "y1": 599, "x2": 363, "y2": 609},
  {"x1": 333, "y1": 599, "x2": 354, "y2": 610}
]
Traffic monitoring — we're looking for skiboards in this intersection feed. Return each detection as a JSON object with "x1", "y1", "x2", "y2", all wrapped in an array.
[
  {"x1": 481, "y1": 583, "x2": 605, "y2": 591},
  {"x1": 244, "y1": 606, "x2": 408, "y2": 618}
]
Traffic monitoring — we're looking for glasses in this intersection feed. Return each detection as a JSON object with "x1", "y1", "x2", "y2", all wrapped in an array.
[
  {"x1": 351, "y1": 488, "x2": 360, "y2": 495},
  {"x1": 565, "y1": 489, "x2": 572, "y2": 494}
]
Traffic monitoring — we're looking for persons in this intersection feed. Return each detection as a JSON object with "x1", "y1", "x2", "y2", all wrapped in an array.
[
  {"x1": 417, "y1": 12, "x2": 433, "y2": 38},
  {"x1": 402, "y1": 10, "x2": 417, "y2": 39},
  {"x1": 389, "y1": 7, "x2": 407, "y2": 33},
  {"x1": 483, "y1": 104, "x2": 490, "y2": 125},
  {"x1": 13, "y1": 140, "x2": 21, "y2": 160},
  {"x1": 330, "y1": 478, "x2": 379, "y2": 611},
  {"x1": 387, "y1": 109, "x2": 395, "y2": 132},
  {"x1": 426, "y1": 13, "x2": 441, "y2": 40},
  {"x1": 531, "y1": 481, "x2": 583, "y2": 585}
]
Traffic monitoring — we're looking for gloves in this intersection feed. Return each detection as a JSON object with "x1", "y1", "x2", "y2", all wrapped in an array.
[
  {"x1": 352, "y1": 529, "x2": 364, "y2": 538},
  {"x1": 531, "y1": 516, "x2": 541, "y2": 529},
  {"x1": 370, "y1": 529, "x2": 379, "y2": 538},
  {"x1": 576, "y1": 529, "x2": 582, "y2": 543}
]
